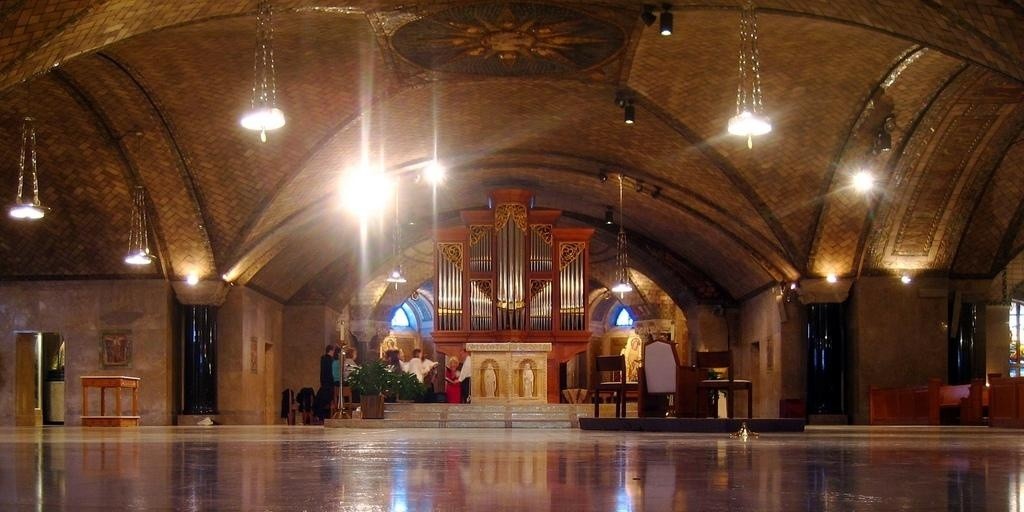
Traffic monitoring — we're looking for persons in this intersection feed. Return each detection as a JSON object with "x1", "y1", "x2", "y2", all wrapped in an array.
[
  {"x1": 344, "y1": 347, "x2": 362, "y2": 382},
  {"x1": 456, "y1": 348, "x2": 472, "y2": 404},
  {"x1": 484, "y1": 363, "x2": 497, "y2": 396},
  {"x1": 319, "y1": 345, "x2": 334, "y2": 390},
  {"x1": 445, "y1": 356, "x2": 462, "y2": 403},
  {"x1": 522, "y1": 363, "x2": 534, "y2": 398},
  {"x1": 333, "y1": 348, "x2": 346, "y2": 385},
  {"x1": 382, "y1": 338, "x2": 439, "y2": 403}
]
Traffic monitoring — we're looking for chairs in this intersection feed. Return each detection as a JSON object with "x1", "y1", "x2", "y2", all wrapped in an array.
[
  {"x1": 592, "y1": 355, "x2": 638, "y2": 416},
  {"x1": 638, "y1": 340, "x2": 710, "y2": 418},
  {"x1": 697, "y1": 349, "x2": 753, "y2": 419},
  {"x1": 284, "y1": 388, "x2": 322, "y2": 425}
]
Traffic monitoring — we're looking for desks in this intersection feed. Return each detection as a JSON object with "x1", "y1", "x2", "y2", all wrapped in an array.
[{"x1": 78, "y1": 375, "x2": 141, "y2": 427}]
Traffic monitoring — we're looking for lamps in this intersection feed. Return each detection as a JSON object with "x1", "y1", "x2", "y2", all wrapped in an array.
[
  {"x1": 8, "y1": 115, "x2": 52, "y2": 228},
  {"x1": 385, "y1": 206, "x2": 408, "y2": 291},
  {"x1": 725, "y1": 3, "x2": 775, "y2": 151},
  {"x1": 238, "y1": 6, "x2": 288, "y2": 145},
  {"x1": 610, "y1": 177, "x2": 634, "y2": 302},
  {"x1": 122, "y1": 176, "x2": 156, "y2": 270}
]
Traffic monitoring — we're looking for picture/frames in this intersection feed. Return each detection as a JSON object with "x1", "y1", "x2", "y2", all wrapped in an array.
[{"x1": 97, "y1": 327, "x2": 134, "y2": 370}]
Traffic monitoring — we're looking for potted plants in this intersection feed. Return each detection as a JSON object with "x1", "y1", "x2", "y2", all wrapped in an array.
[{"x1": 349, "y1": 357, "x2": 396, "y2": 419}]
